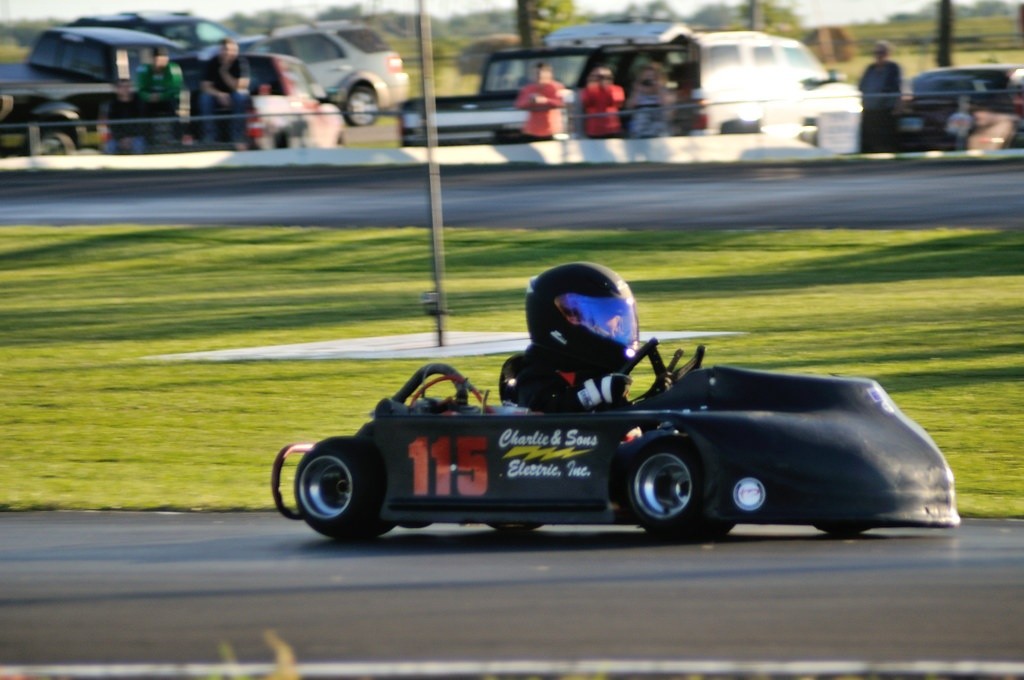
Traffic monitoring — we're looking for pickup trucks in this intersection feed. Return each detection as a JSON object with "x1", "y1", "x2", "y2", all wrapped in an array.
[{"x1": 396, "y1": 37, "x2": 700, "y2": 143}]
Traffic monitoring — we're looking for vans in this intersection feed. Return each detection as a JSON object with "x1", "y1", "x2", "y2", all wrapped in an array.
[{"x1": 694, "y1": 30, "x2": 860, "y2": 134}]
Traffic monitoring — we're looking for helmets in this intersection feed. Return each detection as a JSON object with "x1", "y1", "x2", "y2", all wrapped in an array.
[{"x1": 526, "y1": 261, "x2": 639, "y2": 370}]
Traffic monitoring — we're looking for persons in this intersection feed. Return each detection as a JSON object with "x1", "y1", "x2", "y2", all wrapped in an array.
[
  {"x1": 132, "y1": 47, "x2": 184, "y2": 151},
  {"x1": 515, "y1": 260, "x2": 672, "y2": 417},
  {"x1": 580, "y1": 68, "x2": 625, "y2": 140},
  {"x1": 196, "y1": 36, "x2": 252, "y2": 151},
  {"x1": 625, "y1": 65, "x2": 676, "y2": 139},
  {"x1": 514, "y1": 62, "x2": 569, "y2": 144},
  {"x1": 103, "y1": 78, "x2": 148, "y2": 155},
  {"x1": 859, "y1": 42, "x2": 900, "y2": 152}
]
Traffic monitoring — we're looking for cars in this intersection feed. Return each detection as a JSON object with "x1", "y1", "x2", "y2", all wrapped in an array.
[
  {"x1": 2, "y1": 18, "x2": 414, "y2": 147},
  {"x1": 893, "y1": 65, "x2": 1024, "y2": 143}
]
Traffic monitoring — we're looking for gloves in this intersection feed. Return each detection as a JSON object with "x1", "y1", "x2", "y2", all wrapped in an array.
[{"x1": 569, "y1": 372, "x2": 632, "y2": 414}]
